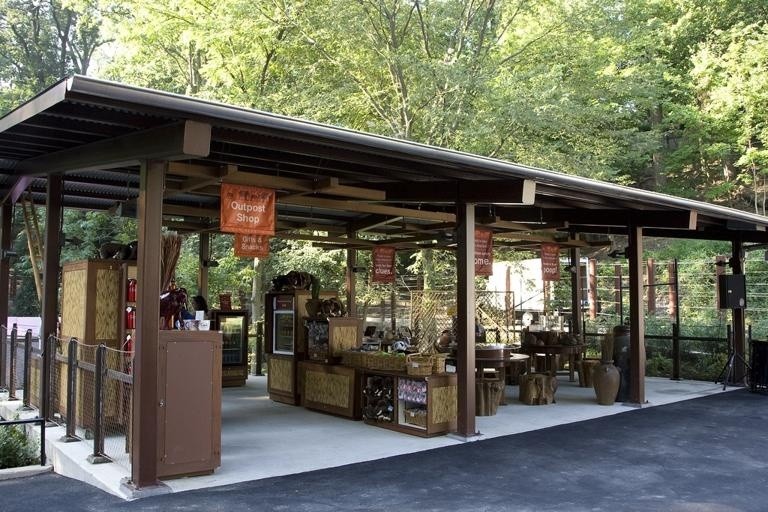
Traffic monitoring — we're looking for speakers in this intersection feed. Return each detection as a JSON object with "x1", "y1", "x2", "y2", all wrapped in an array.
[{"x1": 719, "y1": 274, "x2": 747, "y2": 311}]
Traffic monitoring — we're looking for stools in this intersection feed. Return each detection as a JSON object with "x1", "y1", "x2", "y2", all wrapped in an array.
[
  {"x1": 475, "y1": 378, "x2": 505, "y2": 416},
  {"x1": 575, "y1": 358, "x2": 602, "y2": 387}
]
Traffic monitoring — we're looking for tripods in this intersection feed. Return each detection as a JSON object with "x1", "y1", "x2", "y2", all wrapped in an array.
[{"x1": 715, "y1": 309, "x2": 752, "y2": 392}]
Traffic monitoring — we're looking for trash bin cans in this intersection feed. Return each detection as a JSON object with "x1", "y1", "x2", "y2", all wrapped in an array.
[{"x1": 748, "y1": 337, "x2": 768, "y2": 393}]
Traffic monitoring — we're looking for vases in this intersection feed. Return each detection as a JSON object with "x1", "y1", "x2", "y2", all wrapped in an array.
[
  {"x1": 100, "y1": 242, "x2": 115, "y2": 257},
  {"x1": 592, "y1": 360, "x2": 620, "y2": 405}
]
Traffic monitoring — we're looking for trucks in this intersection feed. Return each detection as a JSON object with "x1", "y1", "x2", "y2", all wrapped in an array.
[{"x1": 487, "y1": 255, "x2": 590, "y2": 325}]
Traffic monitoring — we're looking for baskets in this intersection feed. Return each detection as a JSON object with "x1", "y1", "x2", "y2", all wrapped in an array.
[
  {"x1": 341, "y1": 349, "x2": 406, "y2": 371},
  {"x1": 407, "y1": 353, "x2": 446, "y2": 376}
]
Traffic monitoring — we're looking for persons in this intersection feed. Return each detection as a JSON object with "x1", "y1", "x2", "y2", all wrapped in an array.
[
  {"x1": 192, "y1": 295, "x2": 209, "y2": 320},
  {"x1": 174, "y1": 288, "x2": 194, "y2": 330},
  {"x1": 613, "y1": 325, "x2": 625, "y2": 336}
]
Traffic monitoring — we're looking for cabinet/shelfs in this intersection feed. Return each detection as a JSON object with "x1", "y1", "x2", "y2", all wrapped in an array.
[
  {"x1": 60, "y1": 260, "x2": 124, "y2": 430},
  {"x1": 157, "y1": 329, "x2": 221, "y2": 480},
  {"x1": 264, "y1": 290, "x2": 458, "y2": 436},
  {"x1": 126, "y1": 259, "x2": 137, "y2": 434},
  {"x1": 210, "y1": 308, "x2": 248, "y2": 388}
]
